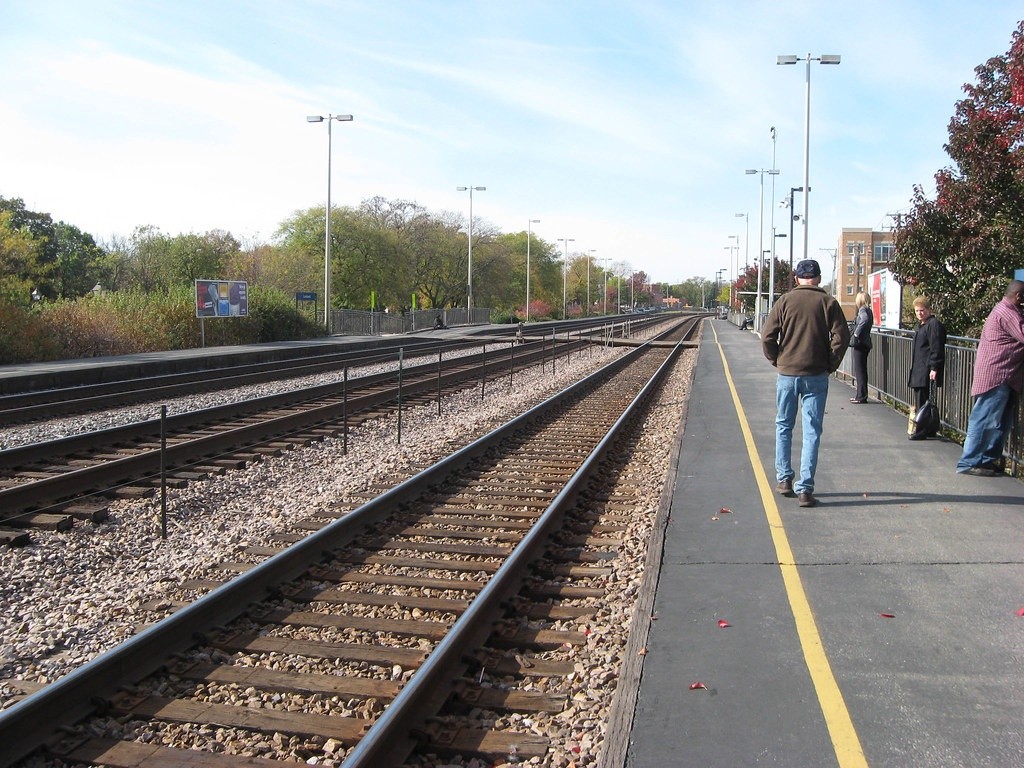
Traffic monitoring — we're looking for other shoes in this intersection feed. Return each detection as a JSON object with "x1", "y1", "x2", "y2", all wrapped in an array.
[
  {"x1": 969, "y1": 465, "x2": 994, "y2": 475},
  {"x1": 908, "y1": 433, "x2": 925, "y2": 440},
  {"x1": 739, "y1": 329, "x2": 743, "y2": 330},
  {"x1": 850, "y1": 398, "x2": 863, "y2": 404}
]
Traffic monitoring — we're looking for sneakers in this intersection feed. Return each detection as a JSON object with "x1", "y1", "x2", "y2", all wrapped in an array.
[
  {"x1": 775, "y1": 481, "x2": 792, "y2": 494},
  {"x1": 798, "y1": 492, "x2": 817, "y2": 506}
]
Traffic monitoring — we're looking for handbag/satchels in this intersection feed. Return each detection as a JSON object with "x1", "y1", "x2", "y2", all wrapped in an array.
[{"x1": 912, "y1": 378, "x2": 940, "y2": 433}]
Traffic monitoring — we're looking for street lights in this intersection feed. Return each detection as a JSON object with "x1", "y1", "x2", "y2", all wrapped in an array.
[
  {"x1": 728, "y1": 235, "x2": 739, "y2": 314},
  {"x1": 762, "y1": 250, "x2": 771, "y2": 292},
  {"x1": 723, "y1": 245, "x2": 740, "y2": 313},
  {"x1": 789, "y1": 185, "x2": 812, "y2": 292},
  {"x1": 627, "y1": 269, "x2": 638, "y2": 312},
  {"x1": 557, "y1": 238, "x2": 575, "y2": 320},
  {"x1": 456, "y1": 184, "x2": 486, "y2": 323},
  {"x1": 776, "y1": 53, "x2": 843, "y2": 261},
  {"x1": 773, "y1": 227, "x2": 788, "y2": 269},
  {"x1": 526, "y1": 219, "x2": 541, "y2": 324},
  {"x1": 600, "y1": 257, "x2": 613, "y2": 315},
  {"x1": 586, "y1": 249, "x2": 597, "y2": 317},
  {"x1": 719, "y1": 269, "x2": 727, "y2": 284},
  {"x1": 618, "y1": 263, "x2": 625, "y2": 315},
  {"x1": 714, "y1": 271, "x2": 722, "y2": 319},
  {"x1": 745, "y1": 167, "x2": 782, "y2": 332},
  {"x1": 304, "y1": 113, "x2": 354, "y2": 336},
  {"x1": 735, "y1": 211, "x2": 749, "y2": 314}
]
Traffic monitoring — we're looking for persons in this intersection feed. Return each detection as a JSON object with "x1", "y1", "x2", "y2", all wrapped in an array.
[
  {"x1": 908, "y1": 297, "x2": 946, "y2": 440},
  {"x1": 956, "y1": 280, "x2": 1024, "y2": 476},
  {"x1": 434, "y1": 315, "x2": 449, "y2": 329},
  {"x1": 761, "y1": 260, "x2": 851, "y2": 506},
  {"x1": 739, "y1": 310, "x2": 755, "y2": 330},
  {"x1": 850, "y1": 291, "x2": 873, "y2": 404}
]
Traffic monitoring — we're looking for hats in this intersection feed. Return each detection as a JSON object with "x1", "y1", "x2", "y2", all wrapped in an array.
[{"x1": 796, "y1": 260, "x2": 820, "y2": 278}]
